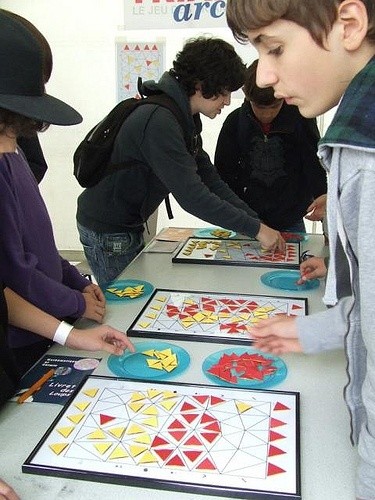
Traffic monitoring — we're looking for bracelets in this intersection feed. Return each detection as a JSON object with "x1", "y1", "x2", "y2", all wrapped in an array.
[{"x1": 53, "y1": 321, "x2": 74, "y2": 345}]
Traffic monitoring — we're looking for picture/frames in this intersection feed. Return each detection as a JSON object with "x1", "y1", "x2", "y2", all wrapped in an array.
[
  {"x1": 22, "y1": 375, "x2": 301, "y2": 499},
  {"x1": 114, "y1": 38, "x2": 166, "y2": 103},
  {"x1": 126, "y1": 288, "x2": 308, "y2": 346},
  {"x1": 171, "y1": 237, "x2": 302, "y2": 269}
]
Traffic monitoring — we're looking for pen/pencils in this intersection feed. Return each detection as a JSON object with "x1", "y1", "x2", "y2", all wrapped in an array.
[{"x1": 17, "y1": 369, "x2": 56, "y2": 404}]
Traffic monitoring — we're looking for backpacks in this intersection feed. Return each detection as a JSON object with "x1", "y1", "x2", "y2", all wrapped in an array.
[{"x1": 73, "y1": 95, "x2": 191, "y2": 188}]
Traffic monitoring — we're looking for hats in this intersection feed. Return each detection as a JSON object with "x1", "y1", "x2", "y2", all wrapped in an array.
[
  {"x1": 0, "y1": 9, "x2": 83, "y2": 126},
  {"x1": 242, "y1": 59, "x2": 277, "y2": 106}
]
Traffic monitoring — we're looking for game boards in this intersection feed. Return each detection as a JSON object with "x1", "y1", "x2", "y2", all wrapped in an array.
[
  {"x1": 124, "y1": 287, "x2": 310, "y2": 351},
  {"x1": 171, "y1": 236, "x2": 304, "y2": 269},
  {"x1": 20, "y1": 374, "x2": 301, "y2": 500}
]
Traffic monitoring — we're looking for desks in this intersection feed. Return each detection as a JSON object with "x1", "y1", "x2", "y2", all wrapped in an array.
[{"x1": 0, "y1": 226, "x2": 357, "y2": 499}]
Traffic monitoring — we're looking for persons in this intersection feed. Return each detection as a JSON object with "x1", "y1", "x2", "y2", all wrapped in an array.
[
  {"x1": 226, "y1": 0, "x2": 375, "y2": 500},
  {"x1": 214, "y1": 58, "x2": 327, "y2": 233},
  {"x1": 0, "y1": 9, "x2": 135, "y2": 500},
  {"x1": 77, "y1": 38, "x2": 287, "y2": 291}
]
